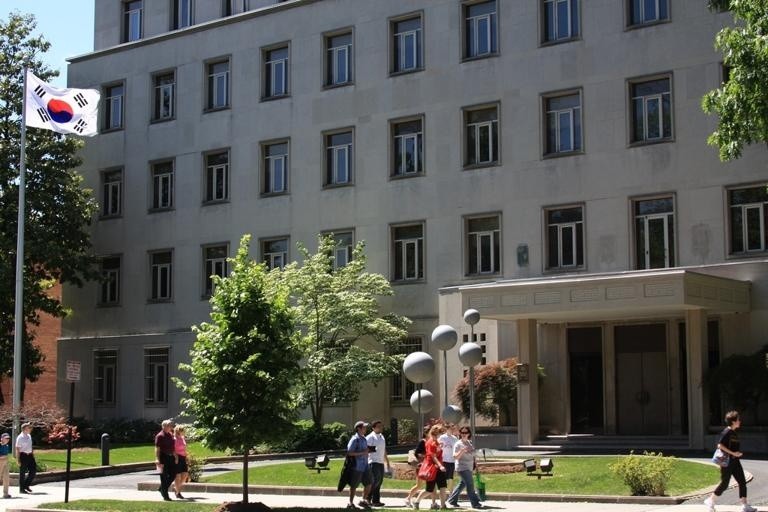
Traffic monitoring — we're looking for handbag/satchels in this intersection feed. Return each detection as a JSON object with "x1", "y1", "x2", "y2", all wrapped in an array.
[
  {"x1": 418, "y1": 462, "x2": 438, "y2": 481},
  {"x1": 712, "y1": 449, "x2": 730, "y2": 467},
  {"x1": 408, "y1": 449, "x2": 419, "y2": 465},
  {"x1": 476, "y1": 480, "x2": 486, "y2": 502}
]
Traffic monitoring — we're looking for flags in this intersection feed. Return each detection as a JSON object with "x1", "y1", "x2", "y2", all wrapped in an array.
[{"x1": 24, "y1": 70, "x2": 100, "y2": 137}]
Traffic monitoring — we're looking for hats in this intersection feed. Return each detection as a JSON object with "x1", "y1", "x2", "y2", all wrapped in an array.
[
  {"x1": 2, "y1": 433, "x2": 10, "y2": 439},
  {"x1": 354, "y1": 421, "x2": 369, "y2": 431}
]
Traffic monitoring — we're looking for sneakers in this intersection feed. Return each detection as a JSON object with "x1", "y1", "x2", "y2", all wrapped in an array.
[
  {"x1": 176, "y1": 493, "x2": 184, "y2": 499},
  {"x1": 24, "y1": 486, "x2": 32, "y2": 492},
  {"x1": 405, "y1": 498, "x2": 413, "y2": 508},
  {"x1": 347, "y1": 503, "x2": 360, "y2": 510},
  {"x1": 20, "y1": 490, "x2": 28, "y2": 494},
  {"x1": 164, "y1": 496, "x2": 171, "y2": 501},
  {"x1": 359, "y1": 499, "x2": 384, "y2": 508},
  {"x1": 431, "y1": 503, "x2": 440, "y2": 509},
  {"x1": 704, "y1": 498, "x2": 715, "y2": 512},
  {"x1": 412, "y1": 499, "x2": 419, "y2": 509},
  {"x1": 742, "y1": 504, "x2": 757, "y2": 512}
]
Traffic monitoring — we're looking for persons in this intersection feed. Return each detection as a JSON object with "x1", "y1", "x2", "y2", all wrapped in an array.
[
  {"x1": 337, "y1": 420, "x2": 377, "y2": 511},
  {"x1": 405, "y1": 422, "x2": 486, "y2": 510},
  {"x1": 170, "y1": 424, "x2": 191, "y2": 498},
  {"x1": 155, "y1": 419, "x2": 179, "y2": 501},
  {"x1": 16, "y1": 423, "x2": 37, "y2": 494},
  {"x1": 0, "y1": 432, "x2": 12, "y2": 498},
  {"x1": 365, "y1": 420, "x2": 393, "y2": 506},
  {"x1": 703, "y1": 411, "x2": 758, "y2": 511}
]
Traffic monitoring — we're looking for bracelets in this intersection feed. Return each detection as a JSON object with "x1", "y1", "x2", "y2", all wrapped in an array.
[{"x1": 174, "y1": 454, "x2": 177, "y2": 458}]
